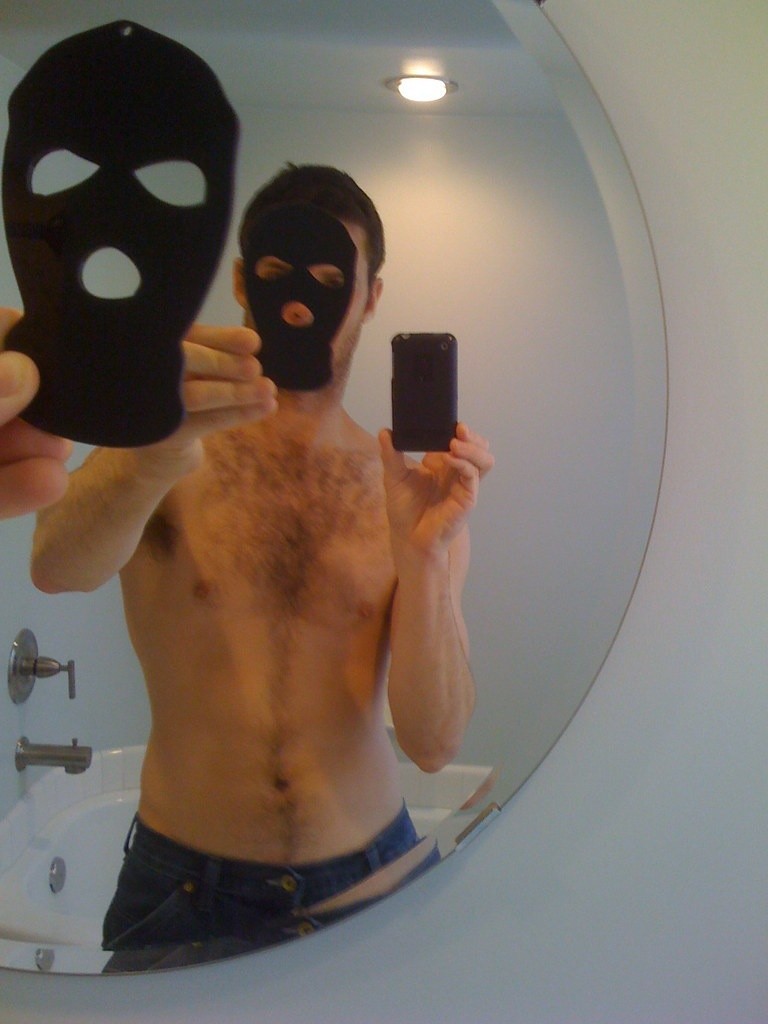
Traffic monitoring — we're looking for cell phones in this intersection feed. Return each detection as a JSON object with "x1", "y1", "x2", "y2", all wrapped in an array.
[{"x1": 390, "y1": 333, "x2": 460, "y2": 452}]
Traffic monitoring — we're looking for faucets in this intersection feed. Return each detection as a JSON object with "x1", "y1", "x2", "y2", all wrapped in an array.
[{"x1": 13, "y1": 734, "x2": 94, "y2": 775}]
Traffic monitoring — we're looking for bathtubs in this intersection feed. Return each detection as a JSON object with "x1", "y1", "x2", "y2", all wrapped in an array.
[{"x1": 0, "y1": 737, "x2": 494, "y2": 980}]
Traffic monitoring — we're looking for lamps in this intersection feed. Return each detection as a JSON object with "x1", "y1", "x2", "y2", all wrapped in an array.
[{"x1": 385, "y1": 64, "x2": 461, "y2": 102}]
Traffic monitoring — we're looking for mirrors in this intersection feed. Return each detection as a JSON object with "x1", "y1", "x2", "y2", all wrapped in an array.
[{"x1": 1, "y1": 0, "x2": 671, "y2": 978}]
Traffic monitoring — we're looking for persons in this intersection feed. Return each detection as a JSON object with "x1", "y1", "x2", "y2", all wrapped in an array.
[
  {"x1": 0, "y1": 306, "x2": 71, "y2": 520},
  {"x1": 28, "y1": 162, "x2": 495, "y2": 974}
]
